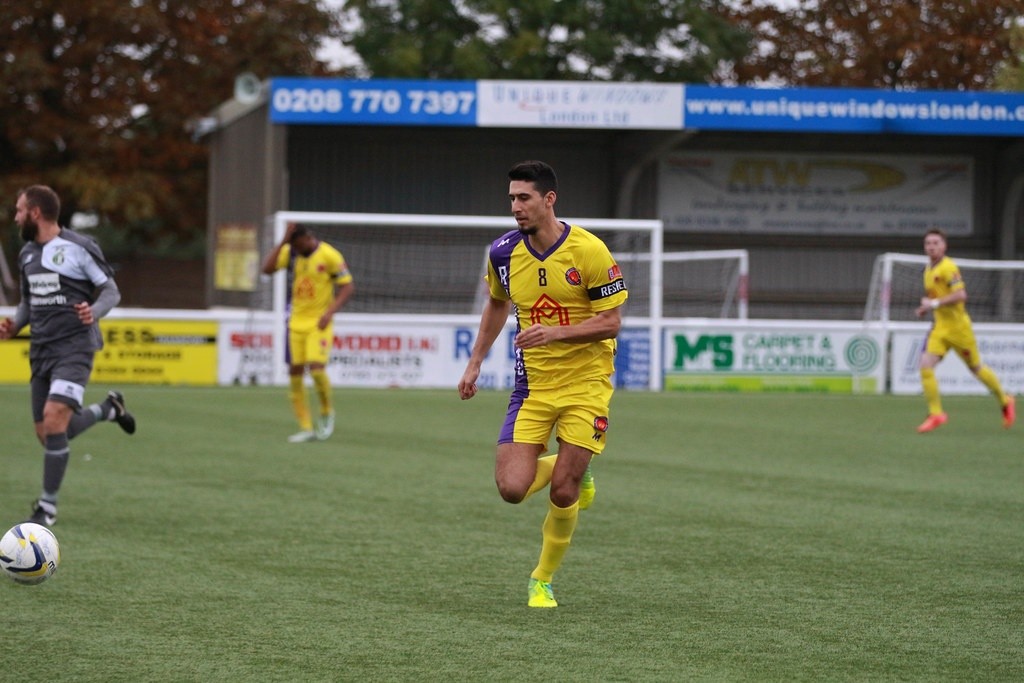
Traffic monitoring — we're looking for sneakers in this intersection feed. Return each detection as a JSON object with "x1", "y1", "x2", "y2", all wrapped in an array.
[
  {"x1": 1000, "y1": 397, "x2": 1014, "y2": 424},
  {"x1": 918, "y1": 413, "x2": 945, "y2": 432},
  {"x1": 526, "y1": 577, "x2": 557, "y2": 608},
  {"x1": 317, "y1": 408, "x2": 334, "y2": 439},
  {"x1": 288, "y1": 430, "x2": 313, "y2": 443},
  {"x1": 29, "y1": 498, "x2": 59, "y2": 526},
  {"x1": 578, "y1": 451, "x2": 594, "y2": 508},
  {"x1": 106, "y1": 390, "x2": 136, "y2": 434}
]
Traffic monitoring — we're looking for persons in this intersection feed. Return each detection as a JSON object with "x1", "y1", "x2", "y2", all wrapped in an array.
[
  {"x1": 915, "y1": 229, "x2": 1016, "y2": 433},
  {"x1": 457, "y1": 160, "x2": 628, "y2": 607},
  {"x1": 0, "y1": 185, "x2": 136, "y2": 527},
  {"x1": 263, "y1": 223, "x2": 354, "y2": 443}
]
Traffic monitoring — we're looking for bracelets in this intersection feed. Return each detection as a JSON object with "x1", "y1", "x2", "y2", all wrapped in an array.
[{"x1": 932, "y1": 299, "x2": 939, "y2": 308}]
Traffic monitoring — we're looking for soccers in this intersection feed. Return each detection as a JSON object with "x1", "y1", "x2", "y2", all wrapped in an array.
[{"x1": 0, "y1": 522, "x2": 62, "y2": 586}]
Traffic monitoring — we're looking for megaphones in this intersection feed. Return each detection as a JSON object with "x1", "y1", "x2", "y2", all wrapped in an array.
[{"x1": 233, "y1": 72, "x2": 261, "y2": 105}]
